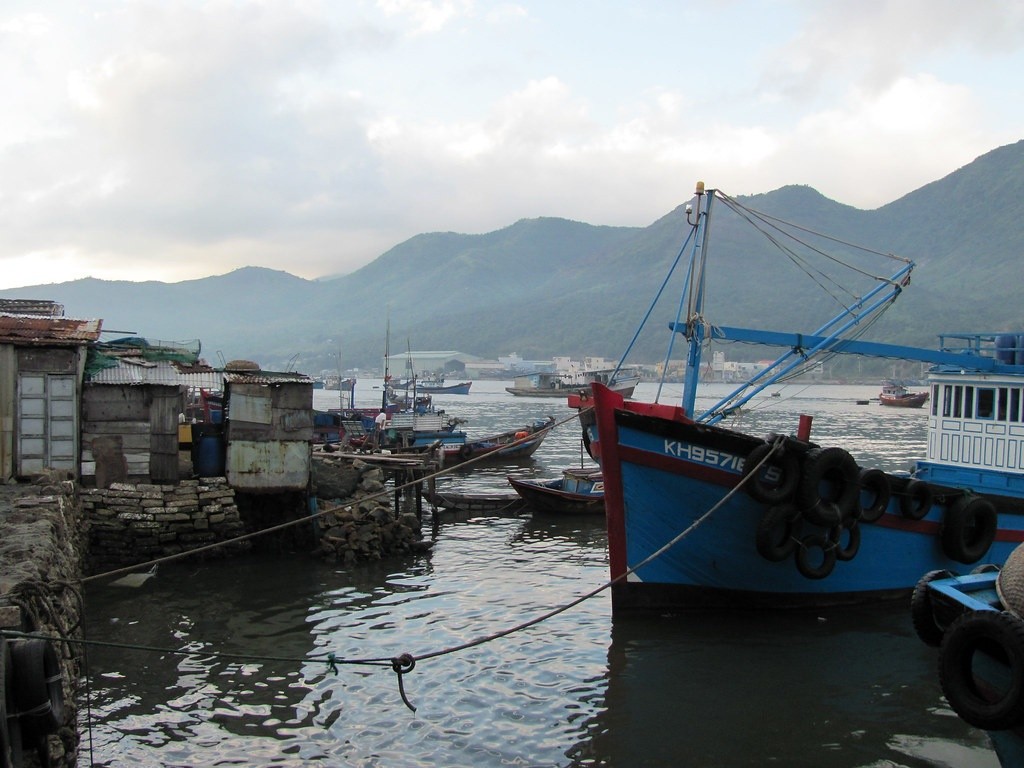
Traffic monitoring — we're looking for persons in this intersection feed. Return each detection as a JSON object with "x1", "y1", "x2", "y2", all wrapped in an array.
[{"x1": 375, "y1": 409, "x2": 386, "y2": 445}]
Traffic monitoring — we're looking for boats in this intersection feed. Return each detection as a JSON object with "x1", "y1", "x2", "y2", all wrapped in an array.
[
  {"x1": 583, "y1": 368, "x2": 640, "y2": 399},
  {"x1": 461, "y1": 417, "x2": 556, "y2": 462},
  {"x1": 561, "y1": 182, "x2": 1024, "y2": 624},
  {"x1": 413, "y1": 370, "x2": 473, "y2": 396},
  {"x1": 302, "y1": 319, "x2": 467, "y2": 469},
  {"x1": 504, "y1": 370, "x2": 594, "y2": 399},
  {"x1": 507, "y1": 467, "x2": 605, "y2": 518},
  {"x1": 880, "y1": 385, "x2": 929, "y2": 409},
  {"x1": 906, "y1": 562, "x2": 1024, "y2": 768},
  {"x1": 311, "y1": 376, "x2": 324, "y2": 389},
  {"x1": 384, "y1": 375, "x2": 416, "y2": 390},
  {"x1": 325, "y1": 374, "x2": 357, "y2": 392}
]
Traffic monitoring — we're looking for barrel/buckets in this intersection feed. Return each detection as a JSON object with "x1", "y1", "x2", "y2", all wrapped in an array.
[{"x1": 191, "y1": 432, "x2": 227, "y2": 477}]
